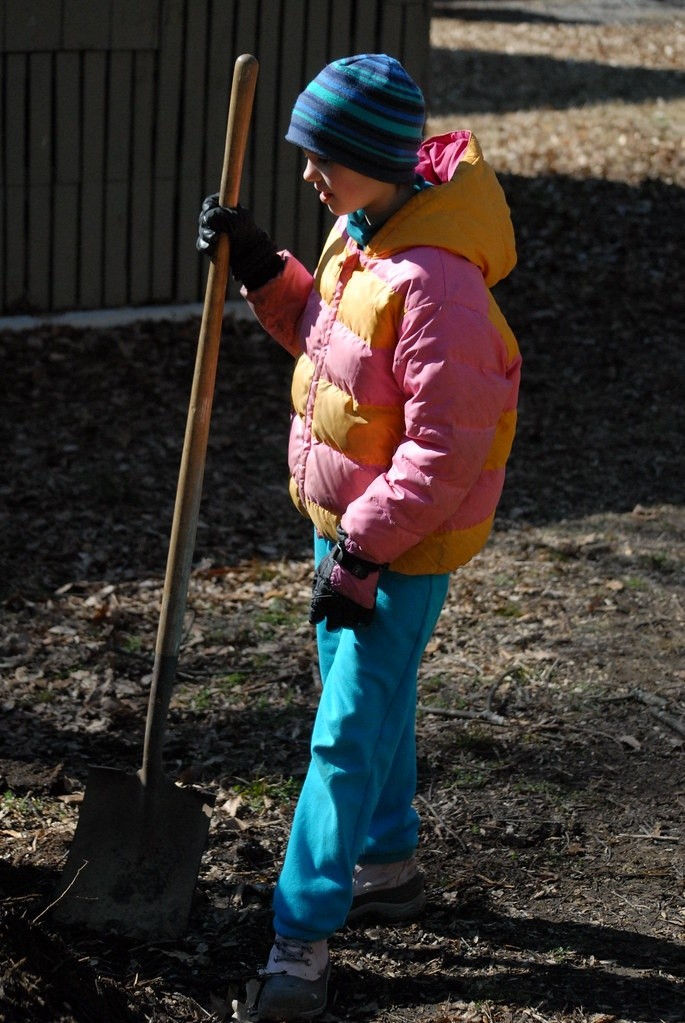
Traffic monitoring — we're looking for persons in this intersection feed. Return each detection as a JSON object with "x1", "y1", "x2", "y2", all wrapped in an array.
[{"x1": 195, "y1": 54, "x2": 523, "y2": 1023}]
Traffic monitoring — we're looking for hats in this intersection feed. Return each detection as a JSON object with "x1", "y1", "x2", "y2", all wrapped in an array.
[{"x1": 284, "y1": 54, "x2": 425, "y2": 185}]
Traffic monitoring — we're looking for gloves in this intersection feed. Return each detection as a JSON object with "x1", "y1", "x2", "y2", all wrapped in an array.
[
  {"x1": 196, "y1": 193, "x2": 285, "y2": 291},
  {"x1": 309, "y1": 524, "x2": 382, "y2": 632}
]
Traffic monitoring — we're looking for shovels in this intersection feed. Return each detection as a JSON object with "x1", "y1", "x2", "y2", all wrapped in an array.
[{"x1": 51, "y1": 51, "x2": 265, "y2": 940}]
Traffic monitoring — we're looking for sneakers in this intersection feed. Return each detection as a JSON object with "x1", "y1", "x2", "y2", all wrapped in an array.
[
  {"x1": 341, "y1": 855, "x2": 427, "y2": 932},
  {"x1": 258, "y1": 937, "x2": 331, "y2": 1023}
]
